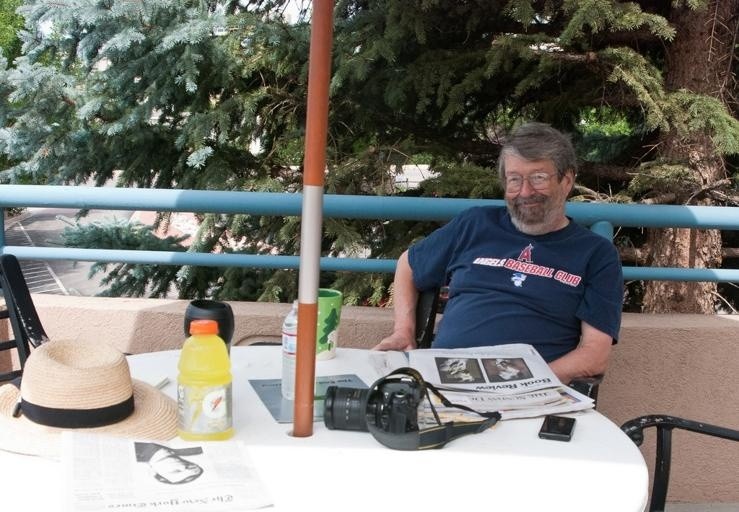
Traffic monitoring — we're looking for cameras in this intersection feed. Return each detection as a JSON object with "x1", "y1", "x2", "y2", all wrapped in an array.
[{"x1": 321, "y1": 374, "x2": 425, "y2": 449}]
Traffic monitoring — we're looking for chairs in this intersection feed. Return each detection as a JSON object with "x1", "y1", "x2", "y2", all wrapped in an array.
[
  {"x1": 0, "y1": 254, "x2": 49, "y2": 388},
  {"x1": 415, "y1": 283, "x2": 605, "y2": 410},
  {"x1": 620, "y1": 414, "x2": 739, "y2": 511}
]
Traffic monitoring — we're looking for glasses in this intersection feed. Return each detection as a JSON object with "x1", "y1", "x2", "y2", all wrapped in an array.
[{"x1": 499, "y1": 170, "x2": 561, "y2": 193}]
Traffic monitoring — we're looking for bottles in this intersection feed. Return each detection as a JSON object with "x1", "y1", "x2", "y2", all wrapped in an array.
[
  {"x1": 278, "y1": 299, "x2": 300, "y2": 402},
  {"x1": 183, "y1": 301, "x2": 233, "y2": 358},
  {"x1": 174, "y1": 318, "x2": 236, "y2": 441}
]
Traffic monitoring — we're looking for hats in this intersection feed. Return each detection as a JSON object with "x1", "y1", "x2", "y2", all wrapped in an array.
[{"x1": 0, "y1": 336, "x2": 180, "y2": 461}]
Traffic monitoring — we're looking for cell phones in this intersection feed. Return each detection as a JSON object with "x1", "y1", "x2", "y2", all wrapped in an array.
[{"x1": 537, "y1": 411, "x2": 578, "y2": 440}]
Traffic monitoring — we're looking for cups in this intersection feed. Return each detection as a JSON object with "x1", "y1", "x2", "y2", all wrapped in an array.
[{"x1": 309, "y1": 289, "x2": 343, "y2": 361}]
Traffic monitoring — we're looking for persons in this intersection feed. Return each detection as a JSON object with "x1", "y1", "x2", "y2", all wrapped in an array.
[
  {"x1": 134, "y1": 441, "x2": 204, "y2": 485},
  {"x1": 371, "y1": 121, "x2": 625, "y2": 386}
]
